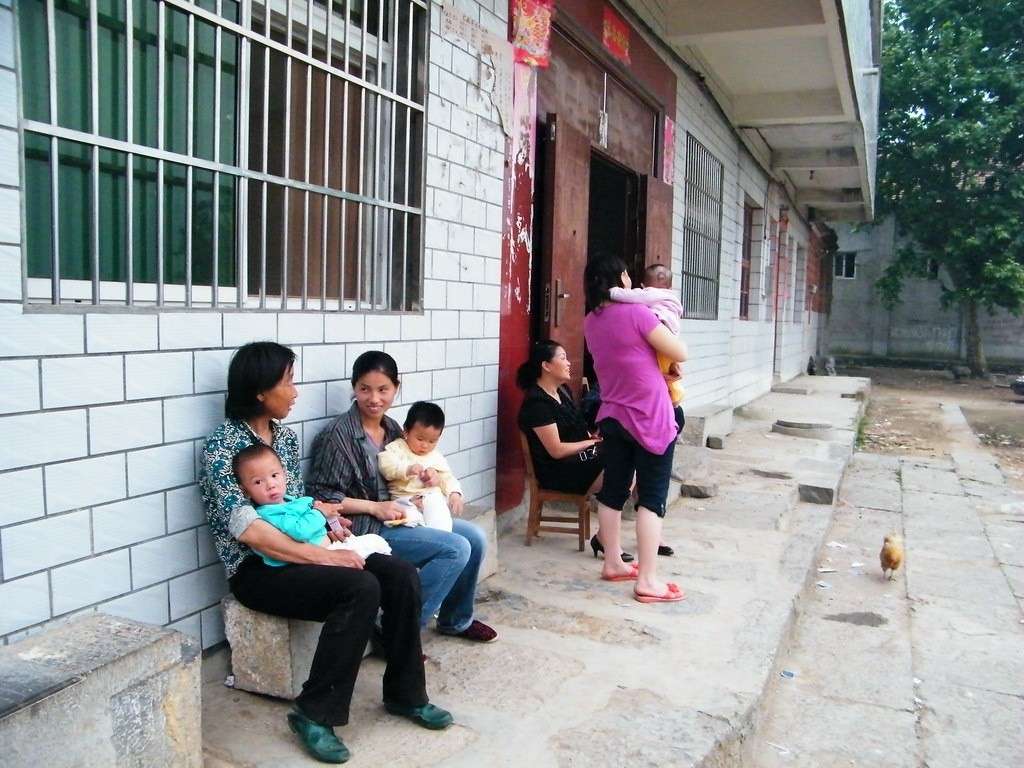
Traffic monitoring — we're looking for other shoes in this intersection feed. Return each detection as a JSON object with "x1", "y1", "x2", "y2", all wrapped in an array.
[
  {"x1": 372, "y1": 646, "x2": 428, "y2": 665},
  {"x1": 456, "y1": 619, "x2": 499, "y2": 643},
  {"x1": 285, "y1": 711, "x2": 350, "y2": 763},
  {"x1": 384, "y1": 701, "x2": 454, "y2": 730}
]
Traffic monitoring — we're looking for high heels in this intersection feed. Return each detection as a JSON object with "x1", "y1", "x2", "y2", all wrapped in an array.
[
  {"x1": 657, "y1": 546, "x2": 674, "y2": 555},
  {"x1": 590, "y1": 535, "x2": 634, "y2": 561}
]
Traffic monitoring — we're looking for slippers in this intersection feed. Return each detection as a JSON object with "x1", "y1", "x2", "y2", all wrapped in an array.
[
  {"x1": 601, "y1": 563, "x2": 639, "y2": 581},
  {"x1": 634, "y1": 583, "x2": 687, "y2": 603}
]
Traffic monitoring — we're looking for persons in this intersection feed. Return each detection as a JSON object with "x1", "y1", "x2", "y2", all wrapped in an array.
[
  {"x1": 609, "y1": 264, "x2": 686, "y2": 433},
  {"x1": 582, "y1": 251, "x2": 687, "y2": 601},
  {"x1": 310, "y1": 351, "x2": 498, "y2": 664},
  {"x1": 201, "y1": 341, "x2": 454, "y2": 763},
  {"x1": 232, "y1": 445, "x2": 391, "y2": 567},
  {"x1": 514, "y1": 339, "x2": 674, "y2": 561},
  {"x1": 377, "y1": 401, "x2": 465, "y2": 532}
]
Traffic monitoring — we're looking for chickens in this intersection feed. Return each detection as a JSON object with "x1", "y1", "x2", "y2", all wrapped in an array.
[{"x1": 879, "y1": 533, "x2": 905, "y2": 582}]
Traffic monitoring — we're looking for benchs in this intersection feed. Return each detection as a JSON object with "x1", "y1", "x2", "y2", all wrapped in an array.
[
  {"x1": 0, "y1": 613, "x2": 204, "y2": 768},
  {"x1": 676, "y1": 403, "x2": 734, "y2": 447},
  {"x1": 221, "y1": 504, "x2": 498, "y2": 697}
]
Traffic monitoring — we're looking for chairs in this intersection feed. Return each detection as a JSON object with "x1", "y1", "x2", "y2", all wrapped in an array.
[{"x1": 518, "y1": 431, "x2": 590, "y2": 551}]
